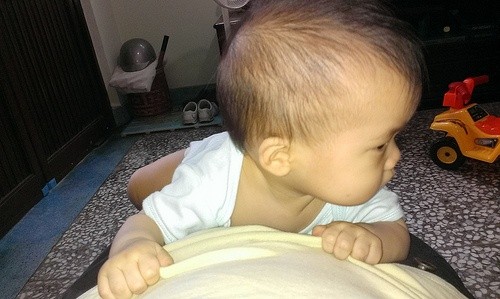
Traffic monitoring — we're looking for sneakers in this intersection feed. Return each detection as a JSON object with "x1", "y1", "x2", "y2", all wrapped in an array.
[
  {"x1": 182, "y1": 101, "x2": 196, "y2": 126},
  {"x1": 199, "y1": 99, "x2": 219, "y2": 122}
]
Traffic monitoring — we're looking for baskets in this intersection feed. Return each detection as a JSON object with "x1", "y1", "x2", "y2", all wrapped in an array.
[{"x1": 116, "y1": 62, "x2": 173, "y2": 116}]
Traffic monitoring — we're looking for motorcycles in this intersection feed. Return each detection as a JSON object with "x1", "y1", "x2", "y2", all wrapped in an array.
[{"x1": 430, "y1": 75, "x2": 500, "y2": 171}]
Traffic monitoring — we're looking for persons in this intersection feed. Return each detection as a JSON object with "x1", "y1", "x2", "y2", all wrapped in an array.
[{"x1": 98, "y1": 0, "x2": 426, "y2": 299}]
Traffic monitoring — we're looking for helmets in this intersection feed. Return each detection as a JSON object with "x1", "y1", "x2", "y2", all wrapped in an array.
[{"x1": 118, "y1": 38, "x2": 157, "y2": 73}]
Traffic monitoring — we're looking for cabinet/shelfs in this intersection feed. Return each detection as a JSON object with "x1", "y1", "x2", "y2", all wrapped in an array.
[{"x1": 379, "y1": 0, "x2": 500, "y2": 110}]
